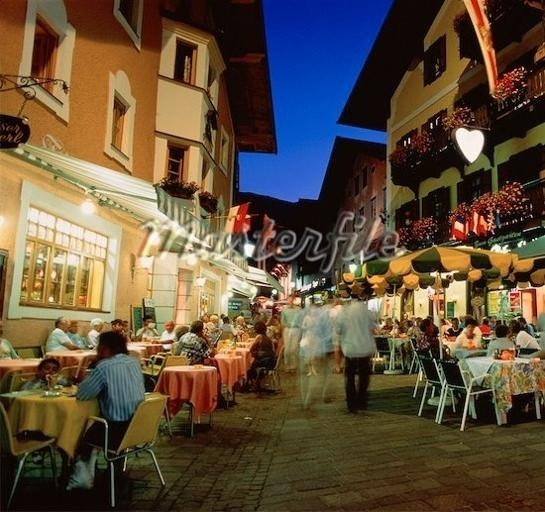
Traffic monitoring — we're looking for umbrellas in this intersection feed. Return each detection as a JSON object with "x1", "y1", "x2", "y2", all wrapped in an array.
[{"x1": 320, "y1": 235, "x2": 545, "y2": 362}]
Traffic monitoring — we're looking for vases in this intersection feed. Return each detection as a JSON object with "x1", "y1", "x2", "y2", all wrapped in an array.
[
  {"x1": 200, "y1": 199, "x2": 218, "y2": 213},
  {"x1": 168, "y1": 188, "x2": 193, "y2": 200}
]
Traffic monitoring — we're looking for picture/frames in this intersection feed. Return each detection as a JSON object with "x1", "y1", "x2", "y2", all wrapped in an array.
[{"x1": 0, "y1": 247, "x2": 10, "y2": 333}]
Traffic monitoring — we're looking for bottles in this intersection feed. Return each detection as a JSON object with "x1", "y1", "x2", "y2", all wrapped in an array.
[
  {"x1": 494, "y1": 348, "x2": 499, "y2": 360},
  {"x1": 516, "y1": 344, "x2": 520, "y2": 354}
]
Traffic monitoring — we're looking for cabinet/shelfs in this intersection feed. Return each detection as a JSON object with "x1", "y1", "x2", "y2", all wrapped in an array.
[{"x1": 20, "y1": 253, "x2": 89, "y2": 307}]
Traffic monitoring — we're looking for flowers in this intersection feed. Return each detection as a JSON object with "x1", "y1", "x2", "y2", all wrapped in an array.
[
  {"x1": 452, "y1": 10, "x2": 478, "y2": 34},
  {"x1": 387, "y1": 145, "x2": 411, "y2": 168},
  {"x1": 199, "y1": 190, "x2": 219, "y2": 205},
  {"x1": 161, "y1": 177, "x2": 200, "y2": 189},
  {"x1": 483, "y1": 0, "x2": 502, "y2": 15},
  {"x1": 395, "y1": 180, "x2": 533, "y2": 250},
  {"x1": 409, "y1": 130, "x2": 433, "y2": 155},
  {"x1": 491, "y1": 65, "x2": 529, "y2": 102},
  {"x1": 441, "y1": 107, "x2": 475, "y2": 139}
]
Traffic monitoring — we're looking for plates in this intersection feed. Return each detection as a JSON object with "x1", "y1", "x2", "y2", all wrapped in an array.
[{"x1": 40, "y1": 392, "x2": 60, "y2": 397}]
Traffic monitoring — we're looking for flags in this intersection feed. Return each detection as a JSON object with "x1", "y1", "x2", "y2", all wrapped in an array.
[
  {"x1": 225, "y1": 204, "x2": 249, "y2": 234},
  {"x1": 241, "y1": 214, "x2": 251, "y2": 234}
]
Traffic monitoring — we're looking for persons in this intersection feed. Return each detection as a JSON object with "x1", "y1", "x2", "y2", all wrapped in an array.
[
  {"x1": 46, "y1": 287, "x2": 545, "y2": 413},
  {"x1": 18, "y1": 358, "x2": 66, "y2": 438},
  {"x1": 0, "y1": 322, "x2": 24, "y2": 359},
  {"x1": 54, "y1": 328, "x2": 146, "y2": 493}
]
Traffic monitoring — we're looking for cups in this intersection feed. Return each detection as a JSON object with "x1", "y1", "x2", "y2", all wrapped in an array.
[
  {"x1": 467, "y1": 339, "x2": 472, "y2": 349},
  {"x1": 508, "y1": 348, "x2": 515, "y2": 360}
]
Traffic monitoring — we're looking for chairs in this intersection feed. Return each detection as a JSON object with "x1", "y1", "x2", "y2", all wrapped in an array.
[{"x1": 373, "y1": 317, "x2": 545, "y2": 431}]
